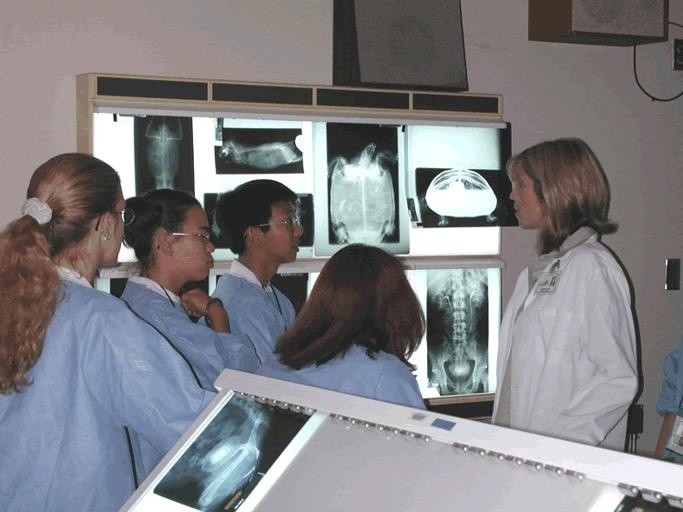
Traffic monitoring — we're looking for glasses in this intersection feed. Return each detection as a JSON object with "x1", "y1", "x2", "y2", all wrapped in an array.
[
  {"x1": 95, "y1": 207, "x2": 136, "y2": 232},
  {"x1": 156, "y1": 230, "x2": 211, "y2": 244},
  {"x1": 242, "y1": 214, "x2": 301, "y2": 233}
]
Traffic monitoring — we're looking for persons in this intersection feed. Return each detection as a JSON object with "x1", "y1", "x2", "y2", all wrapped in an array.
[
  {"x1": 118, "y1": 189, "x2": 259, "y2": 485},
  {"x1": 255, "y1": 241, "x2": 429, "y2": 409},
  {"x1": 0, "y1": 153, "x2": 216, "y2": 511},
  {"x1": 487, "y1": 136, "x2": 640, "y2": 452},
  {"x1": 192, "y1": 180, "x2": 302, "y2": 363}
]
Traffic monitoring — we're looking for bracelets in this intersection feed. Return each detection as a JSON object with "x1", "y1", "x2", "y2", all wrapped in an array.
[{"x1": 204, "y1": 297, "x2": 223, "y2": 326}]
